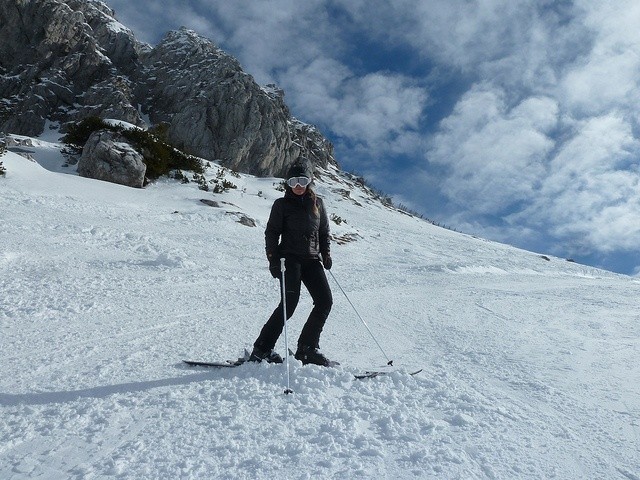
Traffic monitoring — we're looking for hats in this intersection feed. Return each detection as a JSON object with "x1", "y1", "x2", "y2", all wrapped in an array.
[{"x1": 287, "y1": 156, "x2": 314, "y2": 180}]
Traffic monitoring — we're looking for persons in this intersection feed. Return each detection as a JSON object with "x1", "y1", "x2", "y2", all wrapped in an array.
[{"x1": 249, "y1": 167, "x2": 332, "y2": 366}]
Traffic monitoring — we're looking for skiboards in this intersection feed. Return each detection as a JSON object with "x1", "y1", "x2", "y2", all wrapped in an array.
[{"x1": 182, "y1": 361, "x2": 423, "y2": 379}]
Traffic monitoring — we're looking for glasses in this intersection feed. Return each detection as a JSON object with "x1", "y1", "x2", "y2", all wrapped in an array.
[{"x1": 285, "y1": 177, "x2": 312, "y2": 189}]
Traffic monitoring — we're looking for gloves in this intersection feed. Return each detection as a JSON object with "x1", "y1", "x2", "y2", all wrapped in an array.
[
  {"x1": 323, "y1": 254, "x2": 332, "y2": 270},
  {"x1": 268, "y1": 262, "x2": 282, "y2": 279}
]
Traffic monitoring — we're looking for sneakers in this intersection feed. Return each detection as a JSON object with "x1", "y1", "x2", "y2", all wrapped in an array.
[
  {"x1": 248, "y1": 348, "x2": 283, "y2": 363},
  {"x1": 295, "y1": 345, "x2": 330, "y2": 366}
]
